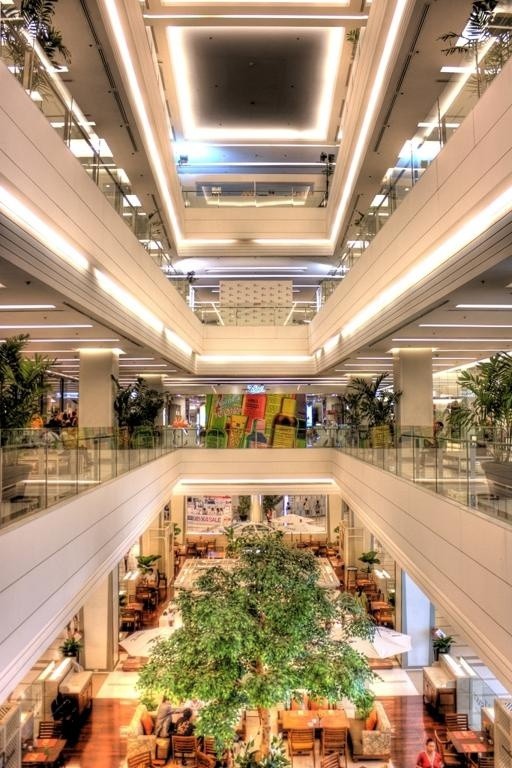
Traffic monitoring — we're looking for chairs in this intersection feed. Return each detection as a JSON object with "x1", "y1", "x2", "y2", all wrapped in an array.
[
  {"x1": 431, "y1": 714, "x2": 494, "y2": 767},
  {"x1": 126, "y1": 701, "x2": 233, "y2": 767},
  {"x1": 22, "y1": 692, "x2": 75, "y2": 767},
  {"x1": 282, "y1": 699, "x2": 391, "y2": 764},
  {"x1": 118, "y1": 537, "x2": 396, "y2": 632}
]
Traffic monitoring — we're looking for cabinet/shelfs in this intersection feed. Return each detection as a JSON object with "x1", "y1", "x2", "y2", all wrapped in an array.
[
  {"x1": 492, "y1": 698, "x2": 512, "y2": 768},
  {"x1": 0, "y1": 703, "x2": 22, "y2": 768}
]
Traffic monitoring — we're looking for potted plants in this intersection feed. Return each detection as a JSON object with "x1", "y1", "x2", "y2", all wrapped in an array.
[
  {"x1": 344, "y1": 369, "x2": 404, "y2": 449},
  {"x1": 110, "y1": 374, "x2": 168, "y2": 449},
  {"x1": 238, "y1": 494, "x2": 250, "y2": 520},
  {"x1": 457, "y1": 351, "x2": 512, "y2": 499},
  {"x1": 0, "y1": 331, "x2": 57, "y2": 500},
  {"x1": 263, "y1": 495, "x2": 282, "y2": 522}
]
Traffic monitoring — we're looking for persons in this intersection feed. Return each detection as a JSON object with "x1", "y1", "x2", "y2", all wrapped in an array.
[
  {"x1": 172, "y1": 707, "x2": 205, "y2": 767},
  {"x1": 189, "y1": 700, "x2": 203, "y2": 707},
  {"x1": 432, "y1": 421, "x2": 449, "y2": 494},
  {"x1": 286, "y1": 502, "x2": 291, "y2": 515},
  {"x1": 414, "y1": 737, "x2": 443, "y2": 768},
  {"x1": 153, "y1": 697, "x2": 185, "y2": 739},
  {"x1": 142, "y1": 568, "x2": 157, "y2": 584},
  {"x1": 303, "y1": 498, "x2": 310, "y2": 518},
  {"x1": 266, "y1": 508, "x2": 272, "y2": 522},
  {"x1": 315, "y1": 500, "x2": 321, "y2": 522},
  {"x1": 42, "y1": 408, "x2": 79, "y2": 449}
]
